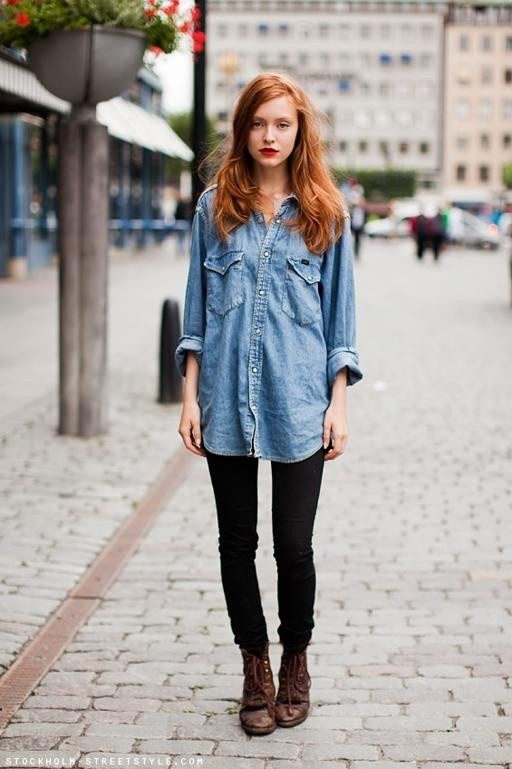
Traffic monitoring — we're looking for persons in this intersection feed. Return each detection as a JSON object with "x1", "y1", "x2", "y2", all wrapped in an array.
[
  {"x1": 351, "y1": 191, "x2": 367, "y2": 257},
  {"x1": 430, "y1": 205, "x2": 447, "y2": 260},
  {"x1": 173, "y1": 71, "x2": 361, "y2": 737},
  {"x1": 411, "y1": 204, "x2": 433, "y2": 258}
]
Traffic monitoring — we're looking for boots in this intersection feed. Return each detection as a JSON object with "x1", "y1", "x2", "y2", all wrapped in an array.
[
  {"x1": 275, "y1": 648, "x2": 310, "y2": 728},
  {"x1": 239, "y1": 642, "x2": 277, "y2": 735}
]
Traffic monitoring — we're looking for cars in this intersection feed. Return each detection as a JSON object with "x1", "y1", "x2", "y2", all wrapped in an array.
[{"x1": 360, "y1": 202, "x2": 503, "y2": 251}]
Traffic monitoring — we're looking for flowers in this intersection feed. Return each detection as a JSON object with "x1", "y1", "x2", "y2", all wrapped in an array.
[{"x1": 0, "y1": 0, "x2": 208, "y2": 67}]
